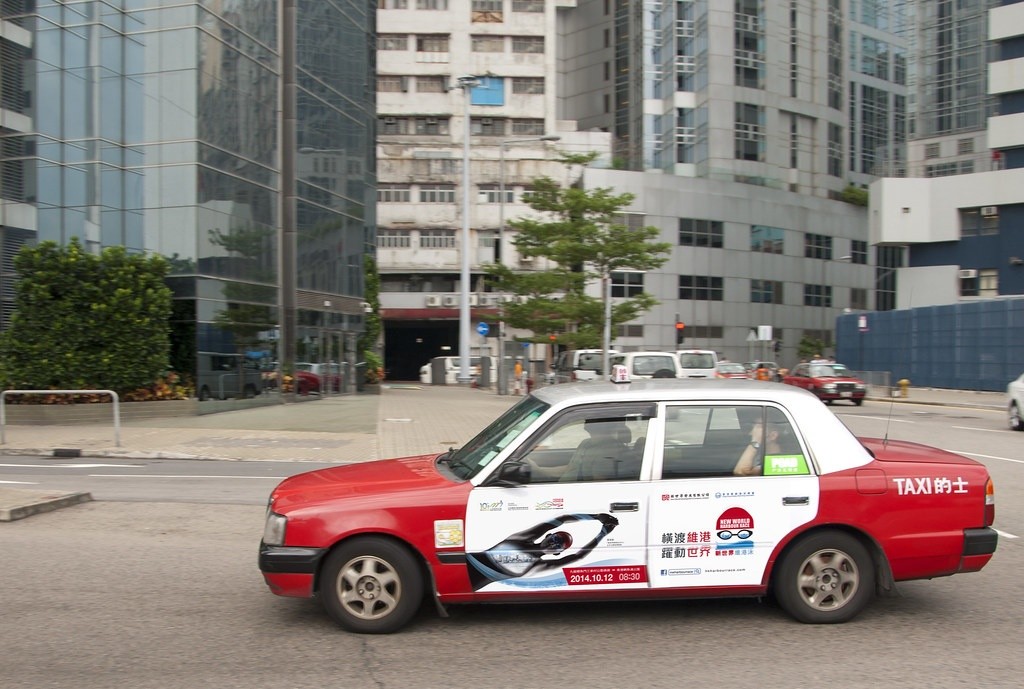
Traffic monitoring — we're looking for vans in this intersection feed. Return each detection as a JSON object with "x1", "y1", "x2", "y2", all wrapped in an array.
[
  {"x1": 550, "y1": 349, "x2": 618, "y2": 385},
  {"x1": 420, "y1": 356, "x2": 498, "y2": 386},
  {"x1": 609, "y1": 352, "x2": 681, "y2": 383},
  {"x1": 671, "y1": 349, "x2": 719, "y2": 378}
]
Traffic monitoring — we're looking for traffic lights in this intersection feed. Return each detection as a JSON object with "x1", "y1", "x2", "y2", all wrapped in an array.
[{"x1": 678, "y1": 330, "x2": 683, "y2": 344}]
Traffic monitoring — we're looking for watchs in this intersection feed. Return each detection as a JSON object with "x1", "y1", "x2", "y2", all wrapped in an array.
[{"x1": 749, "y1": 441, "x2": 760, "y2": 449}]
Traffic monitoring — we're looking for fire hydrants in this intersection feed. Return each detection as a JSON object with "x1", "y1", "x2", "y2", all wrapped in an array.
[{"x1": 899, "y1": 378, "x2": 911, "y2": 398}]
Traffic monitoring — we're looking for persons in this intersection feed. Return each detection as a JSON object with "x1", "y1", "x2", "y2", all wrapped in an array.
[
  {"x1": 512, "y1": 361, "x2": 523, "y2": 396},
  {"x1": 731, "y1": 412, "x2": 785, "y2": 476},
  {"x1": 799, "y1": 353, "x2": 836, "y2": 364},
  {"x1": 527, "y1": 417, "x2": 618, "y2": 481}
]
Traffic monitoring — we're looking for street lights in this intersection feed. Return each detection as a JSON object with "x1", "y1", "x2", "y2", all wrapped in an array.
[
  {"x1": 820, "y1": 255, "x2": 853, "y2": 354},
  {"x1": 499, "y1": 136, "x2": 562, "y2": 394}
]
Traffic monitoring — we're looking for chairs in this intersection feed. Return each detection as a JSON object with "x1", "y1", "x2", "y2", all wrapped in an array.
[{"x1": 618, "y1": 424, "x2": 646, "y2": 478}]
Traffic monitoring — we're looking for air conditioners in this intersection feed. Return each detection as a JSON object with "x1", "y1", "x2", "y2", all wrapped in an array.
[
  {"x1": 981, "y1": 207, "x2": 997, "y2": 216},
  {"x1": 959, "y1": 270, "x2": 978, "y2": 278}
]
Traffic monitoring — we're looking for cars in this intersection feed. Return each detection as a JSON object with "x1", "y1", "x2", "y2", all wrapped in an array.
[
  {"x1": 716, "y1": 362, "x2": 747, "y2": 380},
  {"x1": 1007, "y1": 372, "x2": 1024, "y2": 431},
  {"x1": 781, "y1": 362, "x2": 866, "y2": 405},
  {"x1": 745, "y1": 361, "x2": 781, "y2": 378},
  {"x1": 256, "y1": 376, "x2": 997, "y2": 635}
]
